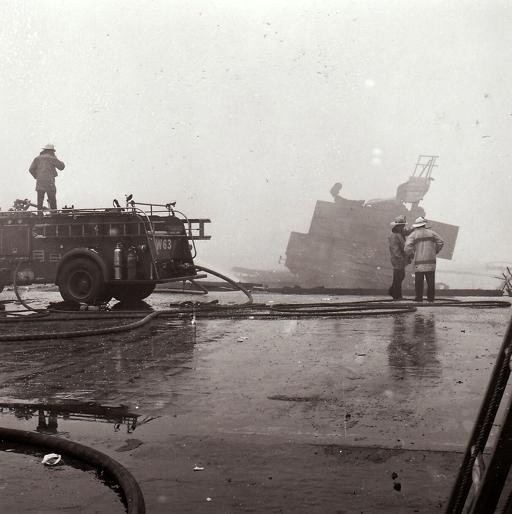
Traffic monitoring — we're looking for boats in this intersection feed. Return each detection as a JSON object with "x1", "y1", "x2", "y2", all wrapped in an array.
[{"x1": 229, "y1": 152, "x2": 461, "y2": 288}]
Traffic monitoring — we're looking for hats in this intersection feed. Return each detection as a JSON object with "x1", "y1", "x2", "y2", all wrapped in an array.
[
  {"x1": 412, "y1": 217, "x2": 427, "y2": 228},
  {"x1": 41, "y1": 143, "x2": 55, "y2": 152},
  {"x1": 390, "y1": 216, "x2": 408, "y2": 225}
]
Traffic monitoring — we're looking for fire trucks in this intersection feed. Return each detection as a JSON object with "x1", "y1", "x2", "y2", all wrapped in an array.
[{"x1": 0, "y1": 196, "x2": 212, "y2": 306}]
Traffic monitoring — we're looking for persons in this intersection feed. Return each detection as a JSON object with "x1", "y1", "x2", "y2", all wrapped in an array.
[
  {"x1": 387, "y1": 214, "x2": 415, "y2": 300},
  {"x1": 403, "y1": 216, "x2": 444, "y2": 302},
  {"x1": 29, "y1": 141, "x2": 66, "y2": 215}
]
